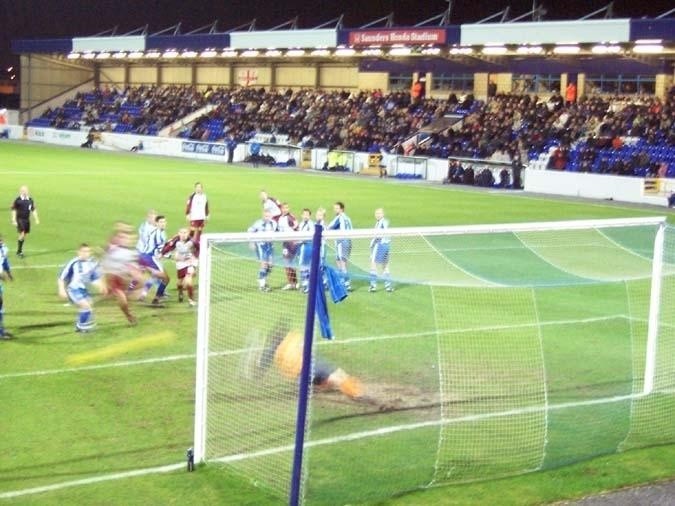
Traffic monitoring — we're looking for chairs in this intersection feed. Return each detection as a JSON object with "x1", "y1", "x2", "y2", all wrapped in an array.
[
  {"x1": 23, "y1": 87, "x2": 255, "y2": 137},
  {"x1": 256, "y1": 87, "x2": 675, "y2": 178}
]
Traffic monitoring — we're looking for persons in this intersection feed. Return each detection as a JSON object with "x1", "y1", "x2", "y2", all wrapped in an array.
[
  {"x1": 236, "y1": 318, "x2": 429, "y2": 415},
  {"x1": 1, "y1": 185, "x2": 40, "y2": 340},
  {"x1": 248, "y1": 191, "x2": 394, "y2": 293},
  {"x1": 45, "y1": 74, "x2": 675, "y2": 191},
  {"x1": 58, "y1": 182, "x2": 211, "y2": 334}
]
